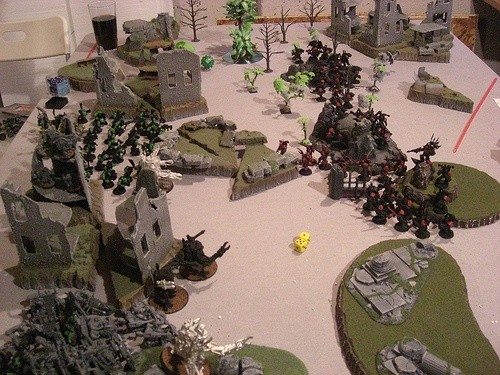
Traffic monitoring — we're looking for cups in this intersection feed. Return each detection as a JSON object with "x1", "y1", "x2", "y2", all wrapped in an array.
[{"x1": 88, "y1": 1, "x2": 118, "y2": 52}]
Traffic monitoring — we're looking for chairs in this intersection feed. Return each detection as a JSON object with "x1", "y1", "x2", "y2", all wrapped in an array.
[{"x1": 0, "y1": 16, "x2": 70, "y2": 159}]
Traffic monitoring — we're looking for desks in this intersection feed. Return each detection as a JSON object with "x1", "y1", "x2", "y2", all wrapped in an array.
[{"x1": 0, "y1": 18, "x2": 499, "y2": 375}]
